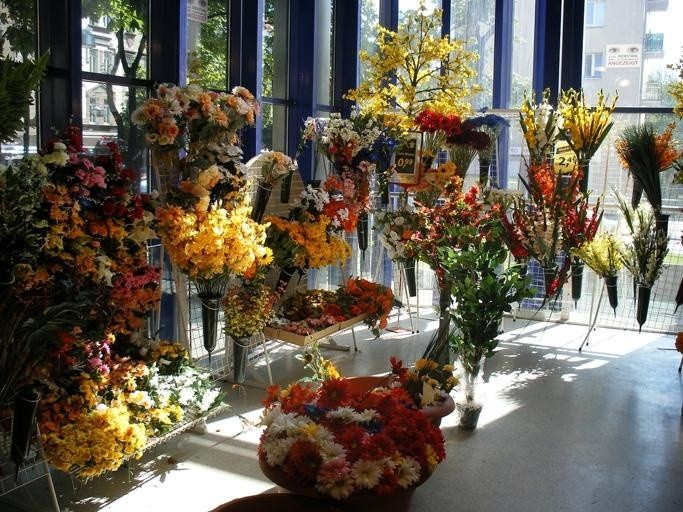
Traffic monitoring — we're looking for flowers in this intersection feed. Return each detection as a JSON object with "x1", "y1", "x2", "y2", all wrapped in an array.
[
  {"x1": 338, "y1": 2, "x2": 486, "y2": 128},
  {"x1": 1, "y1": 82, "x2": 536, "y2": 502},
  {"x1": 519, "y1": 86, "x2": 680, "y2": 327}
]
